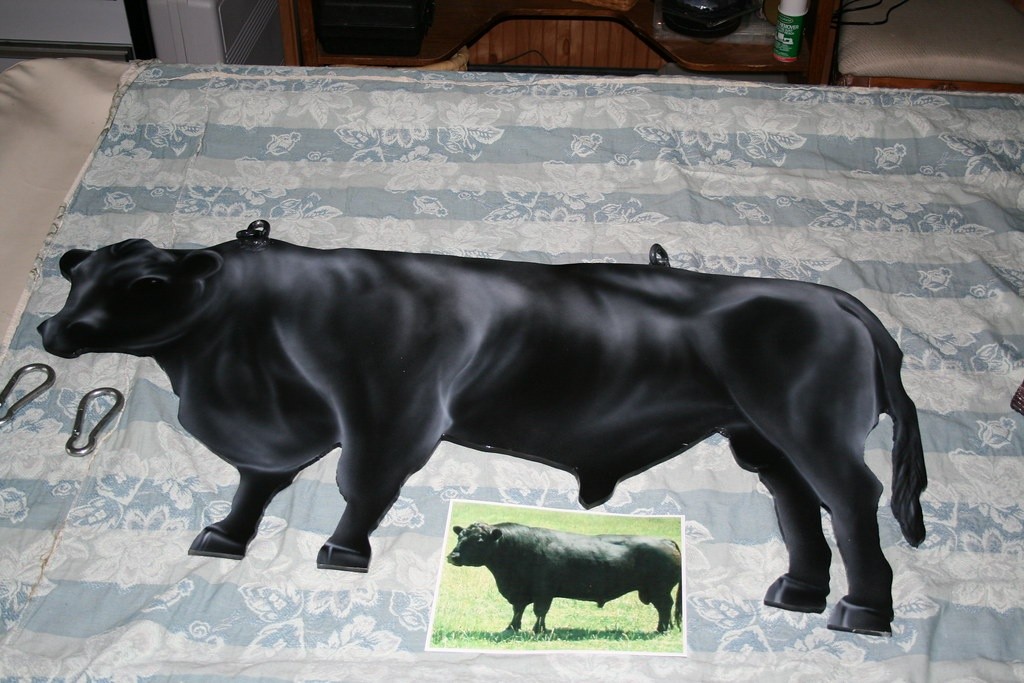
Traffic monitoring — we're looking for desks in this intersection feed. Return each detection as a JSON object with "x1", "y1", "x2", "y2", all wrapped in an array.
[{"x1": 0, "y1": 54, "x2": 1022, "y2": 680}]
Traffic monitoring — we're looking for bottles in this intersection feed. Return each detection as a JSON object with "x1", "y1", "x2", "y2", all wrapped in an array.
[{"x1": 772, "y1": 0, "x2": 808, "y2": 62}]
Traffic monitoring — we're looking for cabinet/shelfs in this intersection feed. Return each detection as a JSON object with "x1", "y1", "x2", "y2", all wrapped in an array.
[{"x1": 276, "y1": 0, "x2": 841, "y2": 86}]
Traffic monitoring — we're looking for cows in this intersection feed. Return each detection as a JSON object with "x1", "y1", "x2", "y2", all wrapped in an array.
[{"x1": 447, "y1": 522, "x2": 681, "y2": 638}]
